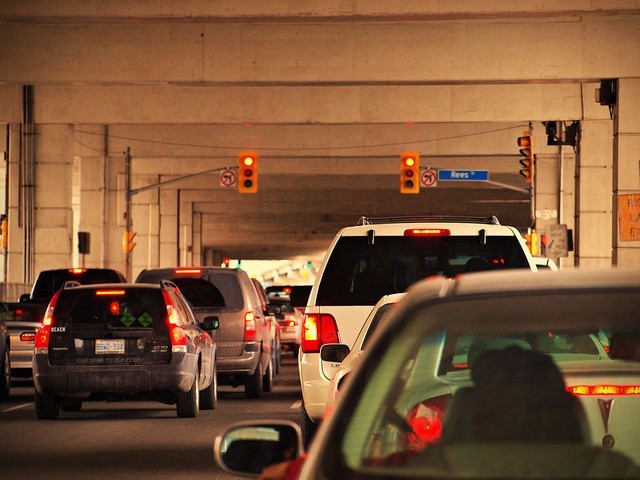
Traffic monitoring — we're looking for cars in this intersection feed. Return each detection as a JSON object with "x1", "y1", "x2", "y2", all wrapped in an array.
[
  {"x1": 133, "y1": 267, "x2": 283, "y2": 397},
  {"x1": 1, "y1": 300, "x2": 44, "y2": 372},
  {"x1": 208, "y1": 261, "x2": 639, "y2": 479},
  {"x1": 30, "y1": 279, "x2": 222, "y2": 417},
  {"x1": 269, "y1": 297, "x2": 304, "y2": 358}
]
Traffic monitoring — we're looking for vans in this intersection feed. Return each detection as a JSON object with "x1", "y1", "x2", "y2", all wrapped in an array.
[
  {"x1": 298, "y1": 213, "x2": 540, "y2": 425},
  {"x1": 19, "y1": 269, "x2": 125, "y2": 312}
]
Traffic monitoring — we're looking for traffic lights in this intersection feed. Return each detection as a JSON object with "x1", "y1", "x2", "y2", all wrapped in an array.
[
  {"x1": 400, "y1": 150, "x2": 419, "y2": 196},
  {"x1": 236, "y1": 144, "x2": 259, "y2": 195},
  {"x1": 127, "y1": 232, "x2": 137, "y2": 253},
  {"x1": 517, "y1": 137, "x2": 530, "y2": 180}
]
